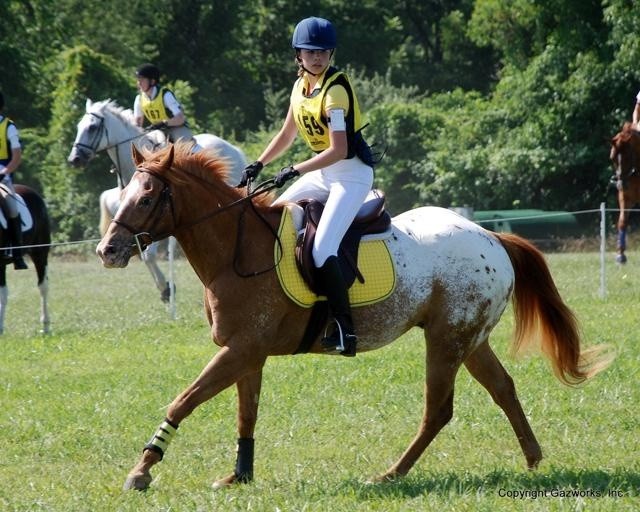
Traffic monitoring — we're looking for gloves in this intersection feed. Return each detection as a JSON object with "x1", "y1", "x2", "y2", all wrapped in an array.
[
  {"x1": 148, "y1": 120, "x2": 168, "y2": 129},
  {"x1": 240, "y1": 161, "x2": 263, "y2": 185},
  {"x1": 273, "y1": 166, "x2": 299, "y2": 187}
]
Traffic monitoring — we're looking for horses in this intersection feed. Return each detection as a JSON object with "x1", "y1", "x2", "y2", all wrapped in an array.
[
  {"x1": 68, "y1": 98, "x2": 249, "y2": 304},
  {"x1": 603, "y1": 122, "x2": 640, "y2": 263},
  {"x1": 95, "y1": 136, "x2": 620, "y2": 491},
  {"x1": 0, "y1": 184, "x2": 53, "y2": 338}
]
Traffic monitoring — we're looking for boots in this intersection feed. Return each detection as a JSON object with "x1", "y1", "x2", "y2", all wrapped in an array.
[
  {"x1": 316, "y1": 255, "x2": 356, "y2": 357},
  {"x1": 8, "y1": 213, "x2": 27, "y2": 270}
]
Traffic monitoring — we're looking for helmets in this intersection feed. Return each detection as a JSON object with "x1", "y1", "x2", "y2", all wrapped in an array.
[
  {"x1": 292, "y1": 16, "x2": 336, "y2": 52},
  {"x1": 137, "y1": 64, "x2": 159, "y2": 78}
]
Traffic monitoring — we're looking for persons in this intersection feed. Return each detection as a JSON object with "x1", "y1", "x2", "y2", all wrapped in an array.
[
  {"x1": 632, "y1": 91, "x2": 640, "y2": 132},
  {"x1": 0, "y1": 112, "x2": 29, "y2": 270},
  {"x1": 132, "y1": 62, "x2": 193, "y2": 143},
  {"x1": 237, "y1": 16, "x2": 376, "y2": 357}
]
